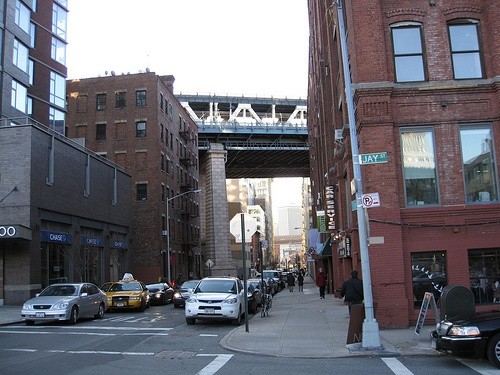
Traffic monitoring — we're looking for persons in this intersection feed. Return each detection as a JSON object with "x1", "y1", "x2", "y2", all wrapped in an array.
[
  {"x1": 340, "y1": 271, "x2": 364, "y2": 315},
  {"x1": 297, "y1": 272, "x2": 304, "y2": 292},
  {"x1": 286, "y1": 273, "x2": 295, "y2": 293},
  {"x1": 316, "y1": 269, "x2": 327, "y2": 300}
]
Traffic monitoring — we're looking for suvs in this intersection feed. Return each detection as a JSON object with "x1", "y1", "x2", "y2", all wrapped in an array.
[{"x1": 184, "y1": 275, "x2": 252, "y2": 326}]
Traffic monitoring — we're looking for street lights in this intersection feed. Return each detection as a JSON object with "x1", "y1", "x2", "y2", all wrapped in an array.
[{"x1": 167, "y1": 189, "x2": 205, "y2": 284}]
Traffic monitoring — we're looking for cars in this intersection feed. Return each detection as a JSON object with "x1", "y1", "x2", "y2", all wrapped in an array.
[
  {"x1": 245, "y1": 268, "x2": 292, "y2": 307},
  {"x1": 145, "y1": 282, "x2": 175, "y2": 306},
  {"x1": 173, "y1": 280, "x2": 201, "y2": 309},
  {"x1": 429, "y1": 309, "x2": 500, "y2": 369},
  {"x1": 20, "y1": 281, "x2": 110, "y2": 326},
  {"x1": 98, "y1": 273, "x2": 151, "y2": 312},
  {"x1": 240, "y1": 280, "x2": 260, "y2": 314}
]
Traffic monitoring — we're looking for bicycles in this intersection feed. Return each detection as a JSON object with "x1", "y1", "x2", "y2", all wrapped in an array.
[{"x1": 260, "y1": 293, "x2": 273, "y2": 318}]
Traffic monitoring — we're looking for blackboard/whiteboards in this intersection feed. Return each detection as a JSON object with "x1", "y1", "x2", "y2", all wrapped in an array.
[{"x1": 414, "y1": 292, "x2": 440, "y2": 336}]
